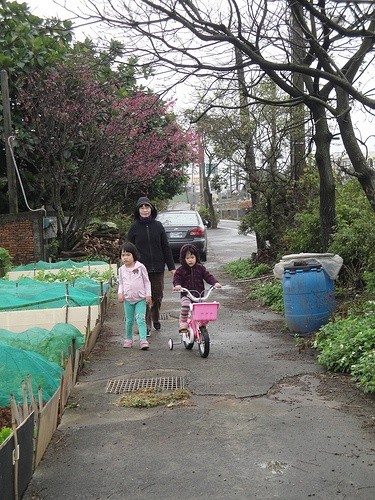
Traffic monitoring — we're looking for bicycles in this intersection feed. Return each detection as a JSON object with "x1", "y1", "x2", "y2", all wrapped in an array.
[{"x1": 166, "y1": 285, "x2": 217, "y2": 358}]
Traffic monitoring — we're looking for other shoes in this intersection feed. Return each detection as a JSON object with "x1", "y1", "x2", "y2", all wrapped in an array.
[
  {"x1": 152, "y1": 321, "x2": 161, "y2": 330},
  {"x1": 147, "y1": 329, "x2": 151, "y2": 337},
  {"x1": 180, "y1": 328, "x2": 187, "y2": 333}
]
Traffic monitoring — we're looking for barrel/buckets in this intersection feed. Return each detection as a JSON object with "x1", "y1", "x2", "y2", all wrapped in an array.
[{"x1": 283, "y1": 262, "x2": 336, "y2": 337}]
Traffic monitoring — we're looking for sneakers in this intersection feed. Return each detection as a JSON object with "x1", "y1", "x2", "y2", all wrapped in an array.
[
  {"x1": 140, "y1": 340, "x2": 149, "y2": 349},
  {"x1": 123, "y1": 340, "x2": 133, "y2": 347}
]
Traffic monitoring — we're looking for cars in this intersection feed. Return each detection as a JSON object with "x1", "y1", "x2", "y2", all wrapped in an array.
[{"x1": 155, "y1": 210, "x2": 209, "y2": 263}]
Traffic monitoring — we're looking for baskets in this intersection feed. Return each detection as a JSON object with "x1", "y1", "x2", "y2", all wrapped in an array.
[{"x1": 193, "y1": 303, "x2": 219, "y2": 322}]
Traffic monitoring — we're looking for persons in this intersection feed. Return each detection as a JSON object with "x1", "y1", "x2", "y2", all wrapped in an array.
[
  {"x1": 172, "y1": 244, "x2": 223, "y2": 344},
  {"x1": 118, "y1": 241, "x2": 151, "y2": 350},
  {"x1": 129, "y1": 197, "x2": 177, "y2": 337}
]
